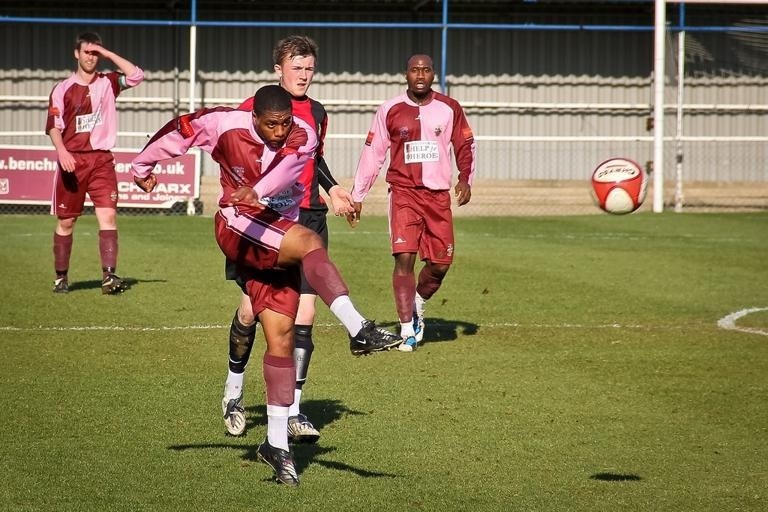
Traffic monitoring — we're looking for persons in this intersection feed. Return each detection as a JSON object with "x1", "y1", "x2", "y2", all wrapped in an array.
[
  {"x1": 220, "y1": 34, "x2": 354, "y2": 445},
  {"x1": 131, "y1": 85, "x2": 403, "y2": 485},
  {"x1": 346, "y1": 52, "x2": 475, "y2": 353},
  {"x1": 45, "y1": 31, "x2": 144, "y2": 296}
]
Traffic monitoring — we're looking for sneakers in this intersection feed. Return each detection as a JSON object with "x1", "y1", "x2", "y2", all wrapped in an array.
[
  {"x1": 257, "y1": 436, "x2": 299, "y2": 486},
  {"x1": 348, "y1": 318, "x2": 404, "y2": 356},
  {"x1": 101, "y1": 275, "x2": 128, "y2": 295},
  {"x1": 413, "y1": 312, "x2": 425, "y2": 343},
  {"x1": 221, "y1": 392, "x2": 246, "y2": 435},
  {"x1": 53, "y1": 275, "x2": 69, "y2": 293},
  {"x1": 399, "y1": 323, "x2": 417, "y2": 352},
  {"x1": 287, "y1": 414, "x2": 320, "y2": 443}
]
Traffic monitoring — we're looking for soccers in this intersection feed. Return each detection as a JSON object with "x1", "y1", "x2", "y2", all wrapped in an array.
[{"x1": 589, "y1": 158, "x2": 649, "y2": 213}]
